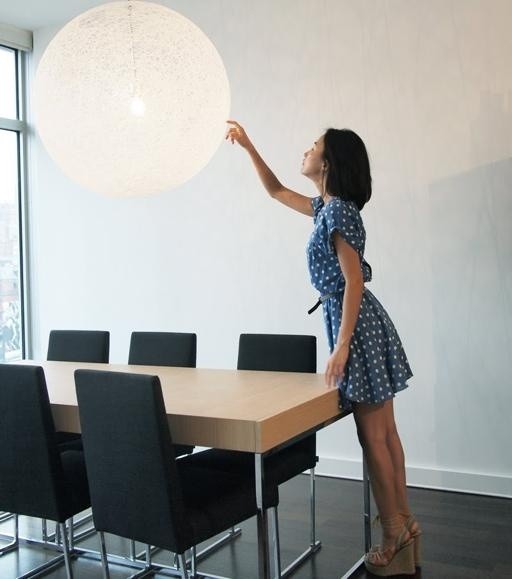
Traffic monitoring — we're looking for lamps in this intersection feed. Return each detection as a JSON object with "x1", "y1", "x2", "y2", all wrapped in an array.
[{"x1": 26, "y1": 0, "x2": 235, "y2": 209}]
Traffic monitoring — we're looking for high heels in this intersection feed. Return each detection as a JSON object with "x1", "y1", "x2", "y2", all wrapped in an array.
[{"x1": 365, "y1": 513, "x2": 423, "y2": 576}]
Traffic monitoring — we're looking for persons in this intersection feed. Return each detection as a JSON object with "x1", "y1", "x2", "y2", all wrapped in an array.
[{"x1": 223, "y1": 120, "x2": 423, "y2": 578}]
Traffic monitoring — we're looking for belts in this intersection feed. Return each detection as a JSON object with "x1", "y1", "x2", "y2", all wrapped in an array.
[{"x1": 308, "y1": 288, "x2": 345, "y2": 314}]
